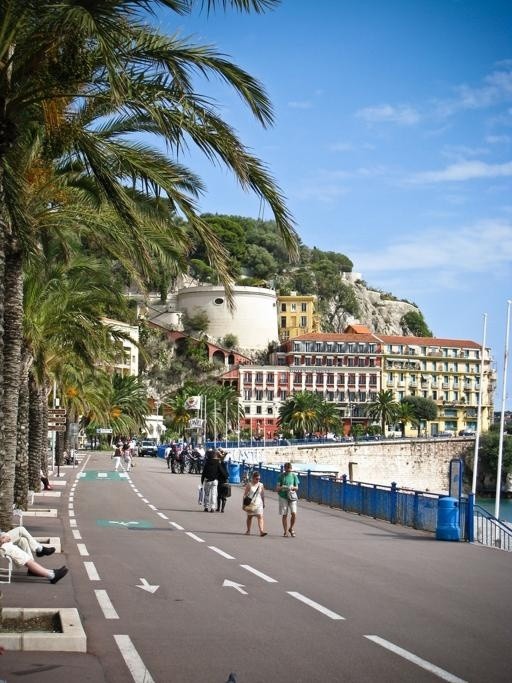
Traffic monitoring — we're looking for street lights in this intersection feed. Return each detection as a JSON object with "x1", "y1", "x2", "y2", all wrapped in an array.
[{"x1": 154, "y1": 401, "x2": 161, "y2": 441}]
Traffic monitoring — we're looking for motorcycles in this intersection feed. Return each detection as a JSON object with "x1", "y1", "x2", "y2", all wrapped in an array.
[{"x1": 241, "y1": 464, "x2": 258, "y2": 486}]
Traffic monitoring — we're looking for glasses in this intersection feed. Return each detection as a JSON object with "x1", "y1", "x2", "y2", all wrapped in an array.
[{"x1": 256, "y1": 478, "x2": 261, "y2": 481}]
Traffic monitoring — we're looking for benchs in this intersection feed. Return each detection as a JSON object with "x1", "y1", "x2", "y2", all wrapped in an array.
[
  {"x1": 12, "y1": 504, "x2": 23, "y2": 528},
  {"x1": 0, "y1": 555, "x2": 13, "y2": 584},
  {"x1": 27, "y1": 491, "x2": 35, "y2": 505}
]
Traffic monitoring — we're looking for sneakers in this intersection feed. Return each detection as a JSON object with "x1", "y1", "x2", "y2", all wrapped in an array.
[
  {"x1": 50, "y1": 565, "x2": 68, "y2": 584},
  {"x1": 283, "y1": 527, "x2": 295, "y2": 536},
  {"x1": 36, "y1": 546, "x2": 55, "y2": 557},
  {"x1": 26, "y1": 568, "x2": 45, "y2": 577},
  {"x1": 260, "y1": 533, "x2": 267, "y2": 536},
  {"x1": 188, "y1": 472, "x2": 202, "y2": 474},
  {"x1": 172, "y1": 471, "x2": 183, "y2": 473},
  {"x1": 204, "y1": 507, "x2": 223, "y2": 512}
]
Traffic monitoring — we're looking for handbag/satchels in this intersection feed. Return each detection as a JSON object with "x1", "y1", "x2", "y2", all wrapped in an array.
[
  {"x1": 223, "y1": 483, "x2": 231, "y2": 496},
  {"x1": 288, "y1": 491, "x2": 298, "y2": 501},
  {"x1": 197, "y1": 486, "x2": 203, "y2": 505},
  {"x1": 242, "y1": 496, "x2": 257, "y2": 512}
]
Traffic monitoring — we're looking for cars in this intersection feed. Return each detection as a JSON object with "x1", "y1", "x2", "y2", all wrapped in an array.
[
  {"x1": 433, "y1": 431, "x2": 452, "y2": 437},
  {"x1": 459, "y1": 429, "x2": 475, "y2": 436},
  {"x1": 115, "y1": 441, "x2": 158, "y2": 456}
]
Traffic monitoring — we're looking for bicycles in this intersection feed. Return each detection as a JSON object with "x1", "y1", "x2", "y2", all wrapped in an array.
[{"x1": 167, "y1": 455, "x2": 204, "y2": 474}]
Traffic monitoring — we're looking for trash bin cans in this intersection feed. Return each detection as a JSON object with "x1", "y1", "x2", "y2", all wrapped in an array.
[
  {"x1": 228, "y1": 464, "x2": 240, "y2": 483},
  {"x1": 436, "y1": 497, "x2": 460, "y2": 541}
]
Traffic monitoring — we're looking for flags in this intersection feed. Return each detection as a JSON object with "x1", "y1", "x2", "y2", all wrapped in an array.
[
  {"x1": 183, "y1": 395, "x2": 202, "y2": 410},
  {"x1": 189, "y1": 417, "x2": 204, "y2": 428}
]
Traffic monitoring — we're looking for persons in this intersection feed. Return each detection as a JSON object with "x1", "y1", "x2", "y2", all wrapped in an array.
[
  {"x1": 243, "y1": 471, "x2": 267, "y2": 537},
  {"x1": 112, "y1": 437, "x2": 137, "y2": 471},
  {"x1": 1, "y1": 524, "x2": 70, "y2": 585},
  {"x1": 201, "y1": 449, "x2": 229, "y2": 511},
  {"x1": 166, "y1": 443, "x2": 204, "y2": 474},
  {"x1": 276, "y1": 461, "x2": 301, "y2": 537},
  {"x1": 217, "y1": 448, "x2": 229, "y2": 513},
  {"x1": 64, "y1": 448, "x2": 71, "y2": 463},
  {"x1": 40, "y1": 470, "x2": 53, "y2": 491}
]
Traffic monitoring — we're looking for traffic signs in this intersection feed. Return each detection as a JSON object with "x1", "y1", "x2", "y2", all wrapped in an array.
[
  {"x1": 48, "y1": 425, "x2": 66, "y2": 431},
  {"x1": 47, "y1": 409, "x2": 66, "y2": 414},
  {"x1": 48, "y1": 417, "x2": 66, "y2": 423}
]
